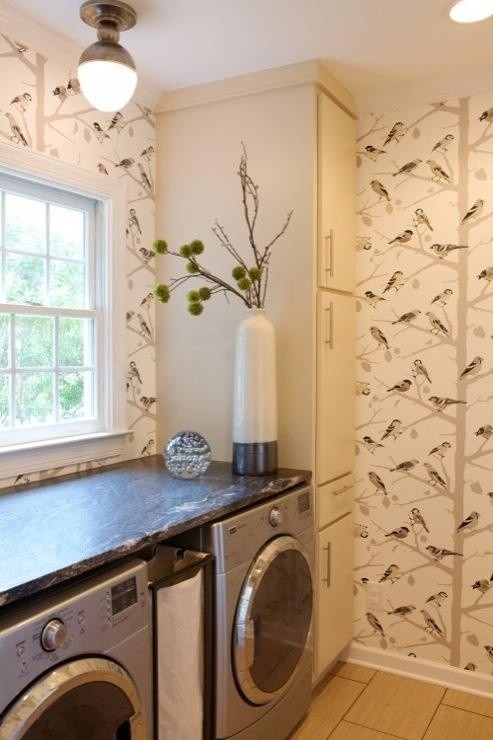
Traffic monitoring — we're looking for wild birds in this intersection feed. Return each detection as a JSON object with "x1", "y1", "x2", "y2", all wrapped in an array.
[
  {"x1": 355, "y1": 106, "x2": 493, "y2": 672},
  {"x1": 4, "y1": 77, "x2": 158, "y2": 458}
]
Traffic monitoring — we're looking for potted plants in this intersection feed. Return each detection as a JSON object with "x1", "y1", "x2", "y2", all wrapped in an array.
[{"x1": 154, "y1": 139, "x2": 297, "y2": 477}]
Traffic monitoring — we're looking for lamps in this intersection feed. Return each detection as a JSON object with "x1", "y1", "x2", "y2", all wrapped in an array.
[{"x1": 77, "y1": 1, "x2": 139, "y2": 111}]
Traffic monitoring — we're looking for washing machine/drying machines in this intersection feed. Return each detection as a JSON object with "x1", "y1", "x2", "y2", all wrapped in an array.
[
  {"x1": 208, "y1": 487, "x2": 322, "y2": 739},
  {"x1": 1, "y1": 556, "x2": 154, "y2": 739}
]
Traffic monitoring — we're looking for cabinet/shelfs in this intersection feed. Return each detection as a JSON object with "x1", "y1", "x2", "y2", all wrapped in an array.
[
  {"x1": 308, "y1": 473, "x2": 357, "y2": 695},
  {"x1": 155, "y1": 290, "x2": 361, "y2": 489},
  {"x1": 157, "y1": 60, "x2": 360, "y2": 296}
]
